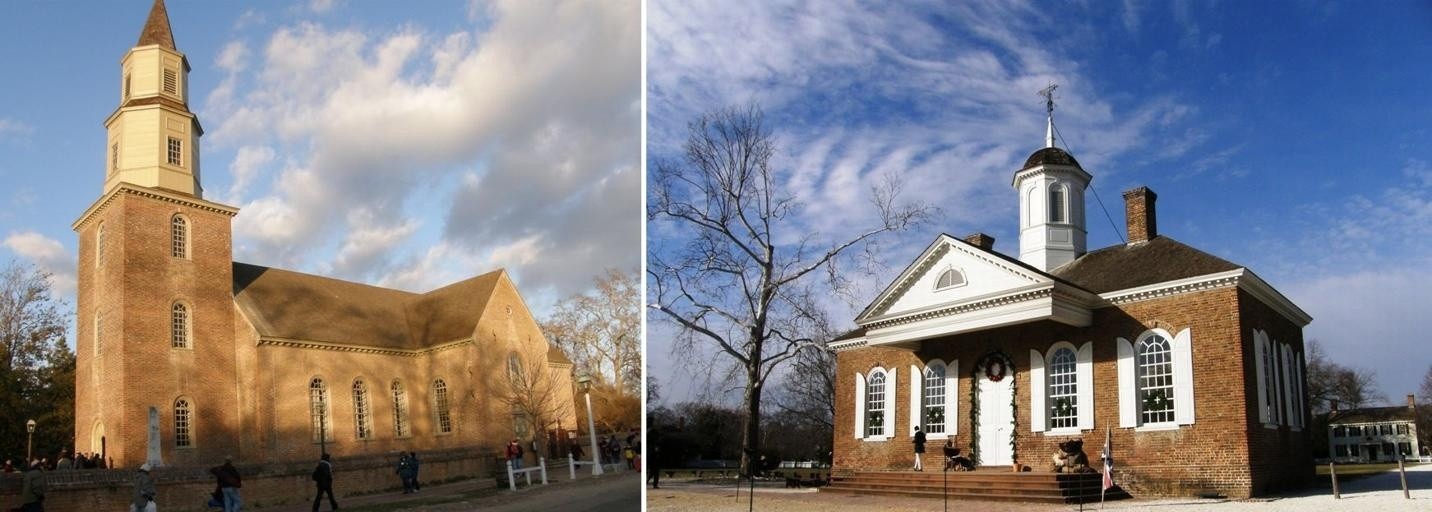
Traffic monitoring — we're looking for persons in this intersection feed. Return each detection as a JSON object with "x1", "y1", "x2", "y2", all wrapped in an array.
[
  {"x1": 217, "y1": 454, "x2": 241, "y2": 512},
  {"x1": 395, "y1": 451, "x2": 414, "y2": 495},
  {"x1": 599, "y1": 433, "x2": 641, "y2": 470},
  {"x1": 571, "y1": 439, "x2": 585, "y2": 469},
  {"x1": 647, "y1": 446, "x2": 662, "y2": 488},
  {"x1": 504, "y1": 440, "x2": 519, "y2": 479},
  {"x1": 912, "y1": 426, "x2": 926, "y2": 472},
  {"x1": 132, "y1": 464, "x2": 157, "y2": 512},
  {"x1": 312, "y1": 455, "x2": 338, "y2": 512},
  {"x1": 3, "y1": 449, "x2": 105, "y2": 473},
  {"x1": 512, "y1": 440, "x2": 524, "y2": 478},
  {"x1": 22, "y1": 459, "x2": 46, "y2": 512},
  {"x1": 410, "y1": 452, "x2": 420, "y2": 490}
]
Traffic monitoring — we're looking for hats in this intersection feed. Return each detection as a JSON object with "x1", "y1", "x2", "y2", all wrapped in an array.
[{"x1": 141, "y1": 464, "x2": 151, "y2": 471}]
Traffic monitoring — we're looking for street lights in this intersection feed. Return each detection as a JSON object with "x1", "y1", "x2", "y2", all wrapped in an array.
[
  {"x1": 24, "y1": 419, "x2": 37, "y2": 469},
  {"x1": 577, "y1": 372, "x2": 606, "y2": 476}
]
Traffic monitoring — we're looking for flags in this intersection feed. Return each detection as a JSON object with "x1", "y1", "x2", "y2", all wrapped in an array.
[{"x1": 1101, "y1": 430, "x2": 1114, "y2": 493}]
